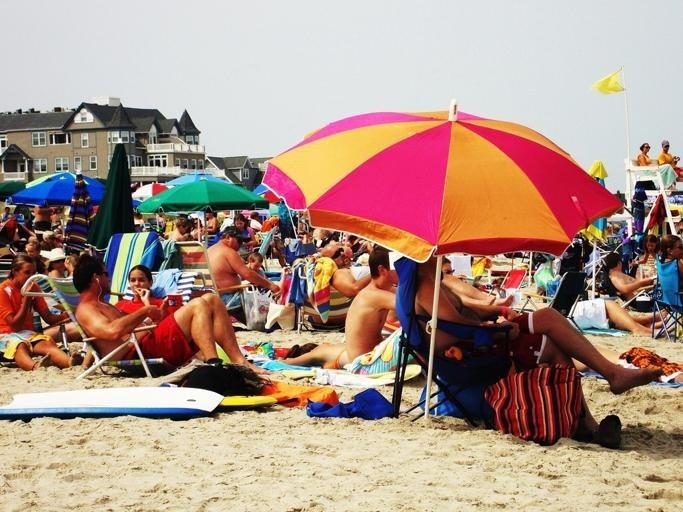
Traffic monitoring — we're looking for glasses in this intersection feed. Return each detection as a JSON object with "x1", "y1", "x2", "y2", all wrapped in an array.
[
  {"x1": 444, "y1": 269, "x2": 455, "y2": 274},
  {"x1": 331, "y1": 248, "x2": 344, "y2": 260},
  {"x1": 664, "y1": 144, "x2": 669, "y2": 148},
  {"x1": 645, "y1": 146, "x2": 650, "y2": 149},
  {"x1": 103, "y1": 272, "x2": 108, "y2": 277}
]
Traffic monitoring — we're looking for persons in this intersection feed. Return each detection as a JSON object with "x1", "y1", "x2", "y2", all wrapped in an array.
[
  {"x1": 638, "y1": 143, "x2": 656, "y2": 166},
  {"x1": 657, "y1": 139, "x2": 683, "y2": 182},
  {"x1": 0, "y1": 208, "x2": 682, "y2": 447}
]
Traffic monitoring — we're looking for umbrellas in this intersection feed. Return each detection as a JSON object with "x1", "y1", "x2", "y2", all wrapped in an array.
[
  {"x1": 130, "y1": 170, "x2": 284, "y2": 221},
  {"x1": 630, "y1": 181, "x2": 647, "y2": 242},
  {"x1": 87, "y1": 138, "x2": 134, "y2": 254},
  {"x1": 5, "y1": 170, "x2": 104, "y2": 206},
  {"x1": 62, "y1": 173, "x2": 94, "y2": 256},
  {"x1": 261, "y1": 101, "x2": 625, "y2": 418},
  {"x1": 577, "y1": 159, "x2": 608, "y2": 298}
]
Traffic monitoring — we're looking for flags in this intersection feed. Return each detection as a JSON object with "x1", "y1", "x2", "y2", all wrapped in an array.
[{"x1": 589, "y1": 66, "x2": 626, "y2": 95}]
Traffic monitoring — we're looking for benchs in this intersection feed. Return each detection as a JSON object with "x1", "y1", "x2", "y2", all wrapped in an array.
[{"x1": 623, "y1": 156, "x2": 683, "y2": 237}]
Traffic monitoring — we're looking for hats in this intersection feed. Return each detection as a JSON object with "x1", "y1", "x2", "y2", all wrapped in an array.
[
  {"x1": 45, "y1": 248, "x2": 67, "y2": 264},
  {"x1": 219, "y1": 226, "x2": 251, "y2": 242}
]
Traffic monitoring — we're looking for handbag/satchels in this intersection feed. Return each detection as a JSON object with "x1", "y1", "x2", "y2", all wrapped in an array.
[
  {"x1": 483, "y1": 359, "x2": 582, "y2": 446},
  {"x1": 264, "y1": 293, "x2": 296, "y2": 330}
]
